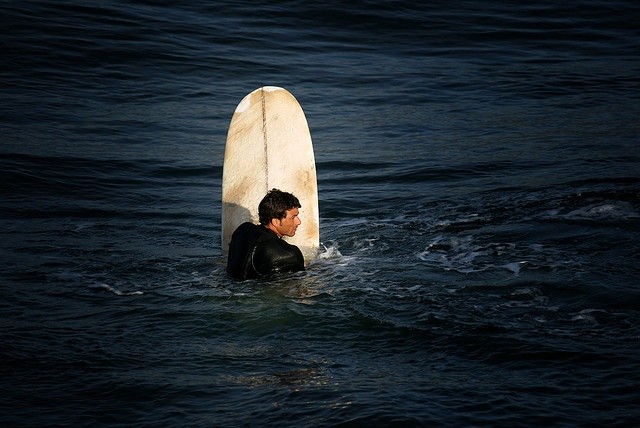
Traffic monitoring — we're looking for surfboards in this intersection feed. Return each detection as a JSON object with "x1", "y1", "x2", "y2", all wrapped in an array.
[{"x1": 219, "y1": 85, "x2": 321, "y2": 261}]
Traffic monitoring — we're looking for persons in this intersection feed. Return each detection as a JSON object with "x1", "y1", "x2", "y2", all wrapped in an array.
[{"x1": 227, "y1": 188, "x2": 304, "y2": 283}]
